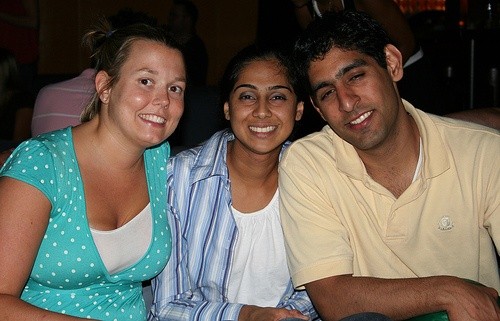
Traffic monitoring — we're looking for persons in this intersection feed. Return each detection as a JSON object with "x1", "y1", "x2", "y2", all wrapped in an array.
[
  {"x1": 278, "y1": 7, "x2": 500, "y2": 321},
  {"x1": 0, "y1": 7, "x2": 187, "y2": 321},
  {"x1": 147, "y1": 40, "x2": 319, "y2": 321},
  {"x1": 0, "y1": 0, "x2": 500, "y2": 173}
]
